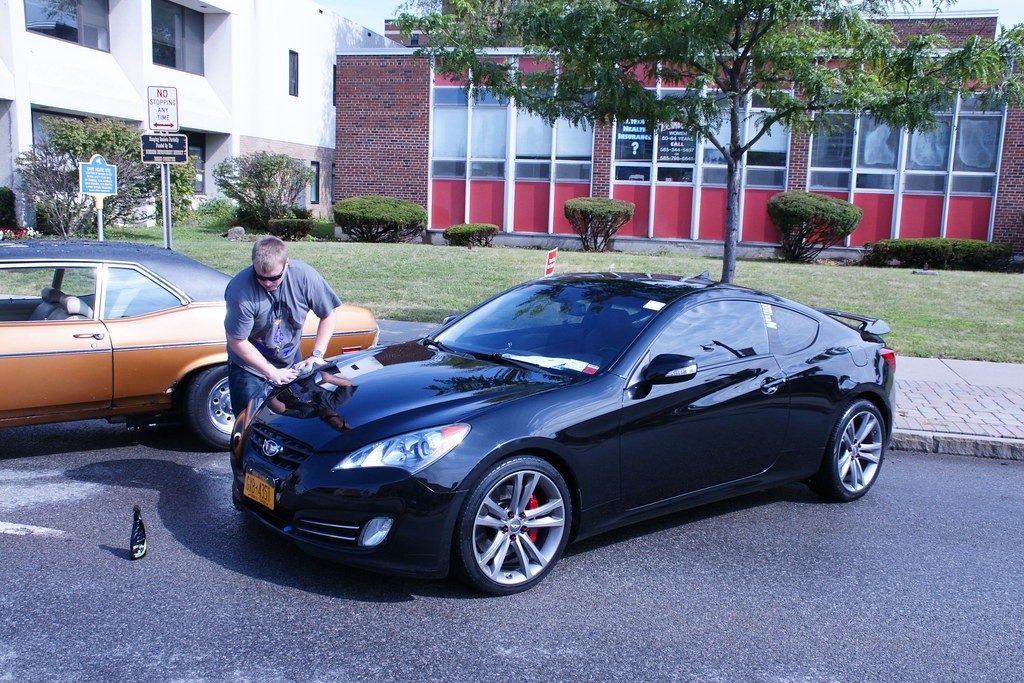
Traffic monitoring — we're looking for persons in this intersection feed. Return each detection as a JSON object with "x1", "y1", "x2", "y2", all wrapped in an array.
[
  {"x1": 222, "y1": 235, "x2": 341, "y2": 413},
  {"x1": 263, "y1": 361, "x2": 356, "y2": 433}
]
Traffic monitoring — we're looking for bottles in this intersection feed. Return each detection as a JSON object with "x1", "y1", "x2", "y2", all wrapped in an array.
[{"x1": 129, "y1": 505, "x2": 147, "y2": 561}]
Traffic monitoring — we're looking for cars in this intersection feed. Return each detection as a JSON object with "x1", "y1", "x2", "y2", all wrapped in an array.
[
  {"x1": 0, "y1": 238, "x2": 381, "y2": 454},
  {"x1": 229, "y1": 270, "x2": 895, "y2": 596}
]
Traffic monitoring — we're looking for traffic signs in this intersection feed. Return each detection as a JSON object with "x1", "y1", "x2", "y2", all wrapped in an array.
[{"x1": 147, "y1": 84, "x2": 180, "y2": 133}]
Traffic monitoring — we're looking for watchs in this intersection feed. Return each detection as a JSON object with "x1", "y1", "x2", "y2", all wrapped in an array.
[{"x1": 309, "y1": 349, "x2": 325, "y2": 360}]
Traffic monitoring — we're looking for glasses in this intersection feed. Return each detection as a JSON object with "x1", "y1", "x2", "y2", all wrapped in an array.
[{"x1": 253, "y1": 264, "x2": 285, "y2": 280}]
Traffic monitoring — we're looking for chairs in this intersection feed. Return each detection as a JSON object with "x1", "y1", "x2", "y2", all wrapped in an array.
[
  {"x1": 585, "y1": 308, "x2": 637, "y2": 359},
  {"x1": 29, "y1": 287, "x2": 65, "y2": 321},
  {"x1": 44, "y1": 295, "x2": 94, "y2": 319}
]
